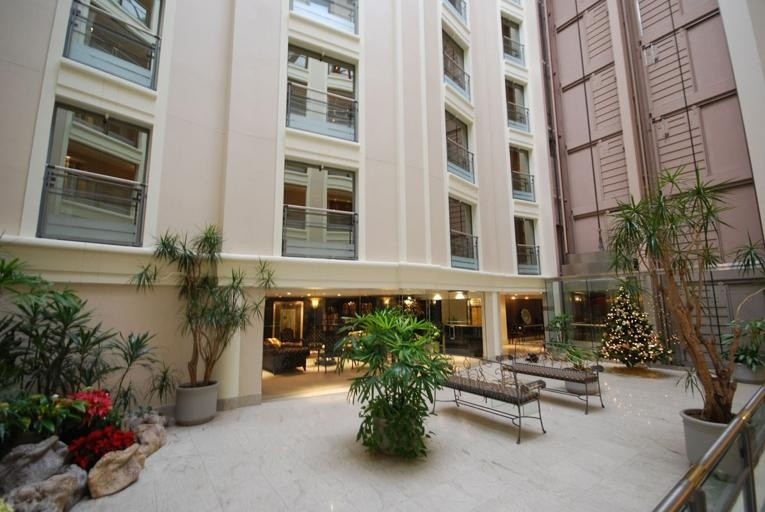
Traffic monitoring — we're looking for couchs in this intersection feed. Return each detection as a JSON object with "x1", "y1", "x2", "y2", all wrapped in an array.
[
  {"x1": 436, "y1": 320, "x2": 484, "y2": 358},
  {"x1": 263, "y1": 334, "x2": 307, "y2": 375}
]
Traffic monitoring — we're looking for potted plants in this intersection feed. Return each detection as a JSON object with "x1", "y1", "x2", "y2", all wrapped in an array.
[
  {"x1": 127, "y1": 224, "x2": 278, "y2": 430},
  {"x1": 714, "y1": 315, "x2": 765, "y2": 384},
  {"x1": 600, "y1": 162, "x2": 765, "y2": 478},
  {"x1": 331, "y1": 310, "x2": 451, "y2": 462},
  {"x1": 549, "y1": 313, "x2": 600, "y2": 393}
]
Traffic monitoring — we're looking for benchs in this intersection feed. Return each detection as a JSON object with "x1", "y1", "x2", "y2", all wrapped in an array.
[
  {"x1": 425, "y1": 352, "x2": 549, "y2": 444},
  {"x1": 498, "y1": 342, "x2": 606, "y2": 414}
]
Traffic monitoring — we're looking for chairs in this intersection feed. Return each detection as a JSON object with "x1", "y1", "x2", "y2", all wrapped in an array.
[
  {"x1": 316, "y1": 333, "x2": 344, "y2": 374},
  {"x1": 505, "y1": 322, "x2": 546, "y2": 343}
]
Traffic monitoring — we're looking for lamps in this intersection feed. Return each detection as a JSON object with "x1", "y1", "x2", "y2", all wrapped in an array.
[{"x1": 308, "y1": 291, "x2": 466, "y2": 312}]
